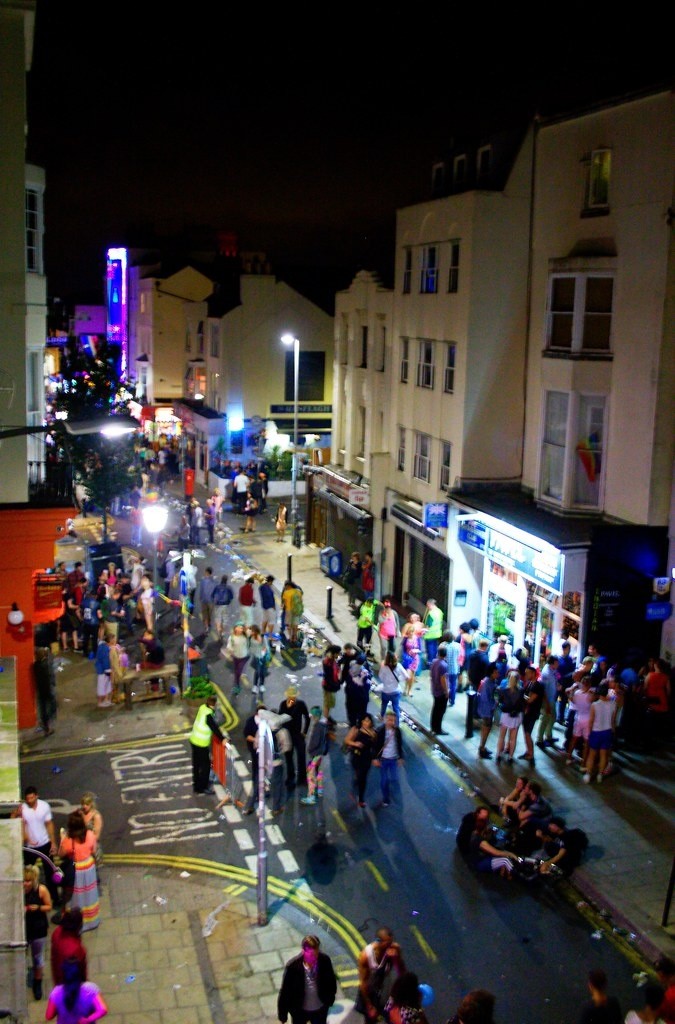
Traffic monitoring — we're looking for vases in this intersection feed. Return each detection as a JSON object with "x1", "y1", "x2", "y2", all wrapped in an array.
[{"x1": 185, "y1": 699, "x2": 208, "y2": 721}]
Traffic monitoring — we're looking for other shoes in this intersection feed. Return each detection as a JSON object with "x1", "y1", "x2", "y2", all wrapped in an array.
[
  {"x1": 326, "y1": 716, "x2": 337, "y2": 730},
  {"x1": 45, "y1": 728, "x2": 55, "y2": 736},
  {"x1": 382, "y1": 798, "x2": 403, "y2": 811},
  {"x1": 231, "y1": 686, "x2": 240, "y2": 694},
  {"x1": 479, "y1": 738, "x2": 614, "y2": 783},
  {"x1": 195, "y1": 780, "x2": 215, "y2": 795},
  {"x1": 349, "y1": 793, "x2": 367, "y2": 807},
  {"x1": 64, "y1": 646, "x2": 97, "y2": 658},
  {"x1": 264, "y1": 776, "x2": 323, "y2": 816},
  {"x1": 431, "y1": 728, "x2": 449, "y2": 735},
  {"x1": 268, "y1": 636, "x2": 278, "y2": 641},
  {"x1": 251, "y1": 685, "x2": 266, "y2": 693},
  {"x1": 98, "y1": 701, "x2": 116, "y2": 707}
]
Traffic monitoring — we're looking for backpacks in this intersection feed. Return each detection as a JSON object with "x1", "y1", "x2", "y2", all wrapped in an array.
[
  {"x1": 565, "y1": 828, "x2": 590, "y2": 867},
  {"x1": 156, "y1": 557, "x2": 170, "y2": 579}
]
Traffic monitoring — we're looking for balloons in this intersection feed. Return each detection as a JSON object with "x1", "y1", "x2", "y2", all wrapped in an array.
[{"x1": 418, "y1": 984, "x2": 434, "y2": 1007}]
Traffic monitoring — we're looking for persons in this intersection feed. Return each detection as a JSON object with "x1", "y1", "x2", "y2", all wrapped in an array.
[
  {"x1": 583, "y1": 958, "x2": 674, "y2": 1024},
  {"x1": 50, "y1": 907, "x2": 87, "y2": 984},
  {"x1": 449, "y1": 990, "x2": 496, "y2": 1024},
  {"x1": 46, "y1": 956, "x2": 106, "y2": 1024},
  {"x1": 277, "y1": 935, "x2": 336, "y2": 1024},
  {"x1": 22, "y1": 866, "x2": 51, "y2": 1001},
  {"x1": 30, "y1": 421, "x2": 668, "y2": 896},
  {"x1": 359, "y1": 926, "x2": 428, "y2": 1024}
]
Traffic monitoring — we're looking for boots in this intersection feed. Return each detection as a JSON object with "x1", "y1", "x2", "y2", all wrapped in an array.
[{"x1": 32, "y1": 977, "x2": 43, "y2": 1000}]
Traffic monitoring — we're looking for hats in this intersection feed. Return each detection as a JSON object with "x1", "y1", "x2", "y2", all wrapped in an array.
[
  {"x1": 91, "y1": 590, "x2": 99, "y2": 595},
  {"x1": 285, "y1": 686, "x2": 300, "y2": 697},
  {"x1": 232, "y1": 620, "x2": 244, "y2": 627}
]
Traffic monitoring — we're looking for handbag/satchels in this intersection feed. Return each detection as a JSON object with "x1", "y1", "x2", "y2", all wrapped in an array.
[
  {"x1": 355, "y1": 986, "x2": 379, "y2": 1015},
  {"x1": 58, "y1": 836, "x2": 75, "y2": 886},
  {"x1": 340, "y1": 725, "x2": 361, "y2": 754},
  {"x1": 291, "y1": 589, "x2": 304, "y2": 615}
]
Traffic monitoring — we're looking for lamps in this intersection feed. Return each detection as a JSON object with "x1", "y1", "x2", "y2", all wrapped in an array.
[{"x1": 8, "y1": 602, "x2": 24, "y2": 633}]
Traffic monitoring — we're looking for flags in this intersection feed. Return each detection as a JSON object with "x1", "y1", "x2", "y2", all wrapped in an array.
[{"x1": 577, "y1": 431, "x2": 601, "y2": 483}]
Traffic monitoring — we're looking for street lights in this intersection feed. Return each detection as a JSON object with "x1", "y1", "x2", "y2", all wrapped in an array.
[{"x1": 280, "y1": 329, "x2": 301, "y2": 524}]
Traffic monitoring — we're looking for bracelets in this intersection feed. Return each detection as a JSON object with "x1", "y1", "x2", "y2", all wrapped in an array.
[{"x1": 37, "y1": 905, "x2": 40, "y2": 910}]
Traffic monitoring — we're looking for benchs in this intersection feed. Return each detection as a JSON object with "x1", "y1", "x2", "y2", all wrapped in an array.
[{"x1": 122, "y1": 664, "x2": 179, "y2": 711}]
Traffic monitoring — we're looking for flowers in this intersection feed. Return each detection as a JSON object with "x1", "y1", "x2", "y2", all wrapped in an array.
[{"x1": 182, "y1": 674, "x2": 216, "y2": 700}]
